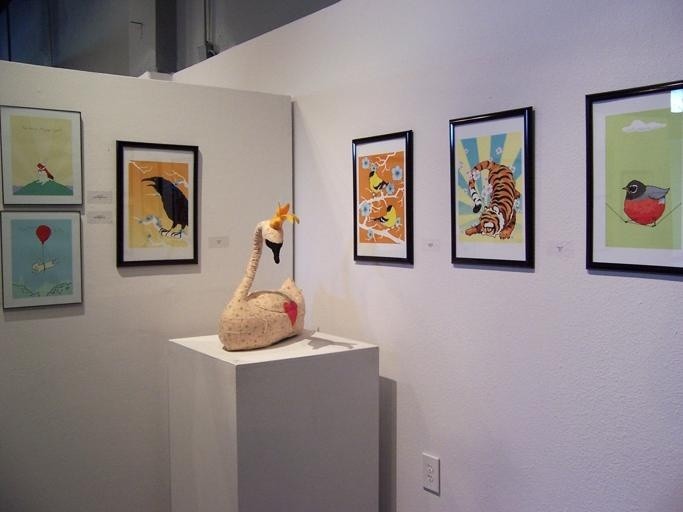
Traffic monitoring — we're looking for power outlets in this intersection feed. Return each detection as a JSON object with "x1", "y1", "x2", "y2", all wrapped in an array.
[{"x1": 419, "y1": 452, "x2": 442, "y2": 494}]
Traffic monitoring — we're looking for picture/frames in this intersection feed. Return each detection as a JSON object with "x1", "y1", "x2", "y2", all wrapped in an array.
[
  {"x1": 0, "y1": 210, "x2": 85, "y2": 310},
  {"x1": 443, "y1": 107, "x2": 537, "y2": 269},
  {"x1": 349, "y1": 129, "x2": 414, "y2": 267},
  {"x1": 114, "y1": 140, "x2": 201, "y2": 270},
  {"x1": 0, "y1": 104, "x2": 83, "y2": 206},
  {"x1": 583, "y1": 80, "x2": 682, "y2": 279}
]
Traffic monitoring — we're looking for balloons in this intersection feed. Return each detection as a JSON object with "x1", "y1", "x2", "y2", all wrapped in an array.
[{"x1": 36, "y1": 225, "x2": 52, "y2": 244}]
